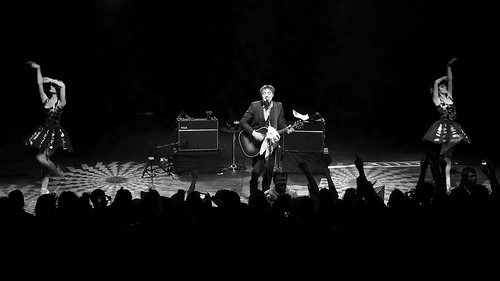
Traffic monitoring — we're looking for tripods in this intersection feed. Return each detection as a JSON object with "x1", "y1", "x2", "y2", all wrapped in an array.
[{"x1": 141, "y1": 142, "x2": 186, "y2": 184}]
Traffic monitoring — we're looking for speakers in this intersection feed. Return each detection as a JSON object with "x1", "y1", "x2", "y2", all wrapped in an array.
[
  {"x1": 282, "y1": 118, "x2": 325, "y2": 152},
  {"x1": 177, "y1": 117, "x2": 218, "y2": 151}
]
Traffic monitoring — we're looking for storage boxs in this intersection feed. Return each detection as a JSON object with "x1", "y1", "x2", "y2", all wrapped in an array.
[
  {"x1": 283, "y1": 118, "x2": 326, "y2": 153},
  {"x1": 175, "y1": 117, "x2": 219, "y2": 151}
]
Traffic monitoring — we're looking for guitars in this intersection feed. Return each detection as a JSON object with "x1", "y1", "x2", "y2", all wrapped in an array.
[{"x1": 237, "y1": 119, "x2": 304, "y2": 158}]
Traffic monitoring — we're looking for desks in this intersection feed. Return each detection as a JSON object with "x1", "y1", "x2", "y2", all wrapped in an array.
[{"x1": 221, "y1": 128, "x2": 248, "y2": 172}]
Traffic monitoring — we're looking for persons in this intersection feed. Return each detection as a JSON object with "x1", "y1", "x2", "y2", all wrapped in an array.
[
  {"x1": 0, "y1": 154, "x2": 500, "y2": 281},
  {"x1": 240, "y1": 84, "x2": 294, "y2": 190},
  {"x1": 27, "y1": 62, "x2": 74, "y2": 196},
  {"x1": 264, "y1": 168, "x2": 298, "y2": 207},
  {"x1": 462, "y1": 167, "x2": 477, "y2": 184},
  {"x1": 423, "y1": 58, "x2": 470, "y2": 191}
]
